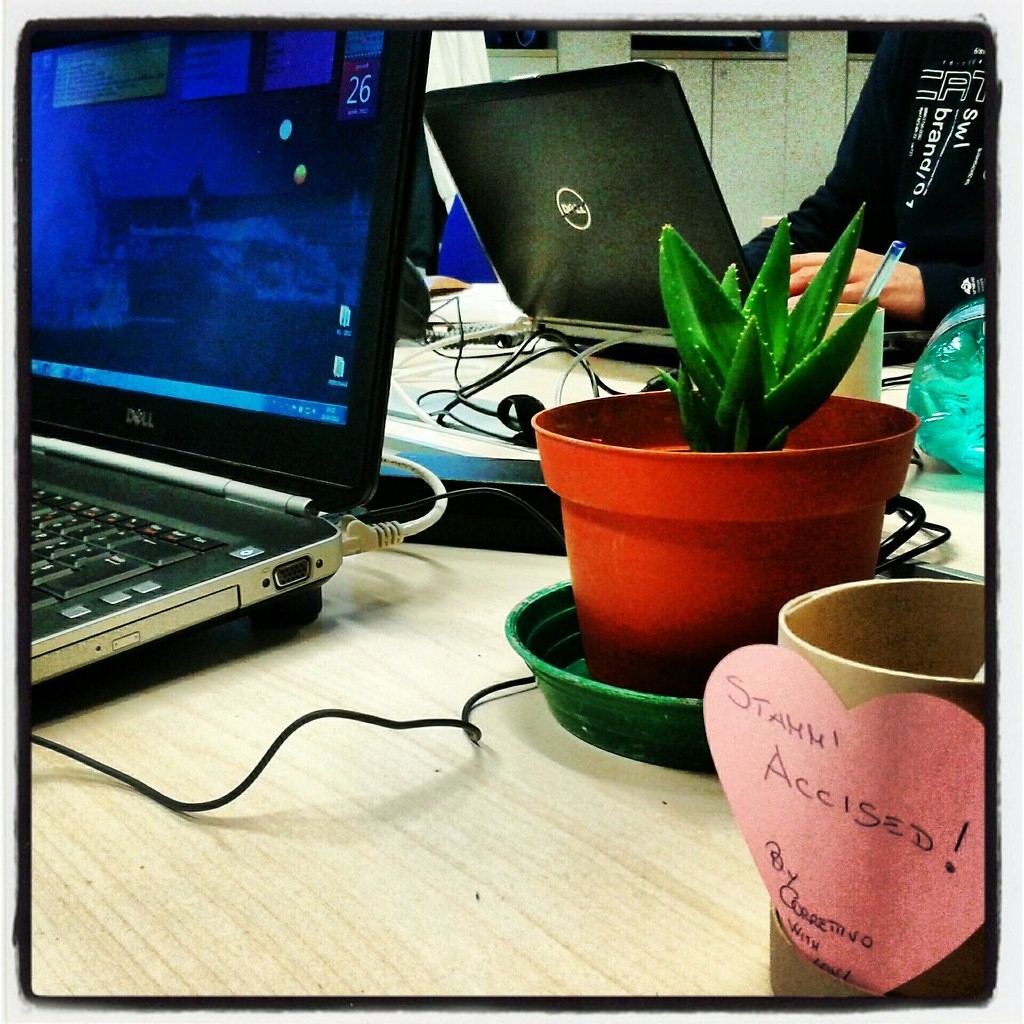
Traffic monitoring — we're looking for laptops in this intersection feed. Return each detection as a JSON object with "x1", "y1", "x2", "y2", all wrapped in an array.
[
  {"x1": 422, "y1": 53, "x2": 933, "y2": 374},
  {"x1": 27, "y1": 25, "x2": 434, "y2": 734}
]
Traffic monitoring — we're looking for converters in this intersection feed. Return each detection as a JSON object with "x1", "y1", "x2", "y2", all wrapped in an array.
[{"x1": 356, "y1": 450, "x2": 566, "y2": 557}]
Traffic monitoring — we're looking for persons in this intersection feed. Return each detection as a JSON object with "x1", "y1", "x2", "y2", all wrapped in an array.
[{"x1": 741, "y1": 20, "x2": 992, "y2": 326}]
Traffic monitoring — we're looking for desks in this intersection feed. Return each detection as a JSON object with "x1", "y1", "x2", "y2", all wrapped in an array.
[{"x1": 33, "y1": 278, "x2": 985, "y2": 997}]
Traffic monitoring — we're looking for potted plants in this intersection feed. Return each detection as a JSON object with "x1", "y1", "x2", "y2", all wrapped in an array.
[{"x1": 503, "y1": 199, "x2": 919, "y2": 785}]
